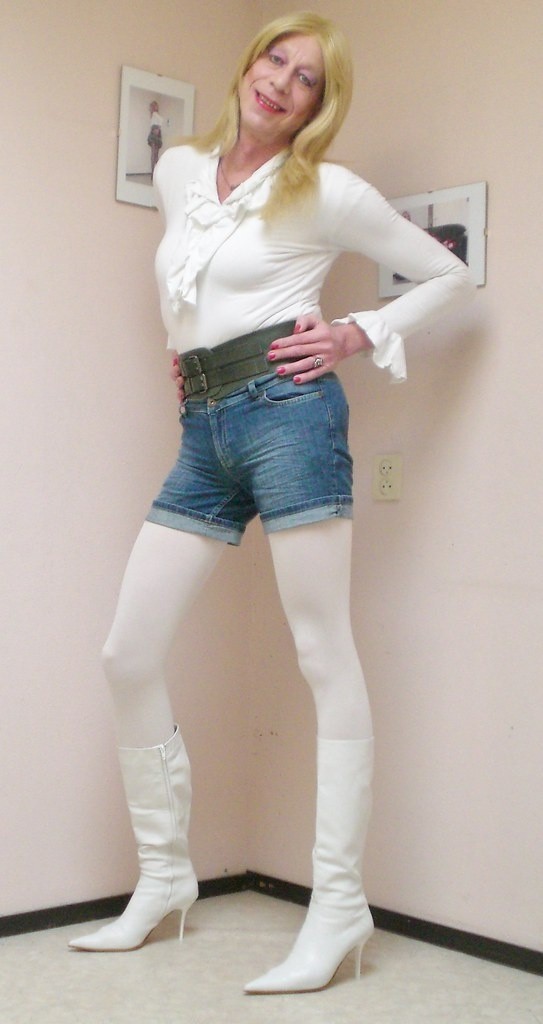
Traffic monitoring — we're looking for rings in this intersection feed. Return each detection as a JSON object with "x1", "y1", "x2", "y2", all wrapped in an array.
[{"x1": 311, "y1": 354, "x2": 323, "y2": 367}]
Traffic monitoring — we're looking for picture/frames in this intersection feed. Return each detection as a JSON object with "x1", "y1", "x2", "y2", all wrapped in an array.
[
  {"x1": 115, "y1": 65, "x2": 196, "y2": 208},
  {"x1": 378, "y1": 179, "x2": 488, "y2": 299}
]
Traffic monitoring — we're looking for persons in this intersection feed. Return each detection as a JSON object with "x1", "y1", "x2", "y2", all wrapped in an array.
[
  {"x1": 147, "y1": 100, "x2": 170, "y2": 179},
  {"x1": 69, "y1": 12, "x2": 475, "y2": 995}
]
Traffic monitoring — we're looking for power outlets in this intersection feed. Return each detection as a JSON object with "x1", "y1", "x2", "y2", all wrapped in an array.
[{"x1": 372, "y1": 454, "x2": 404, "y2": 502}]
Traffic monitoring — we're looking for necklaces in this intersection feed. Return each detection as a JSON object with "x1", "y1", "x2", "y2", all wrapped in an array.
[{"x1": 220, "y1": 161, "x2": 263, "y2": 190}]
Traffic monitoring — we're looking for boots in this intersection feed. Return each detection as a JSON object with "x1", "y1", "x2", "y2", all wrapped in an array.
[
  {"x1": 244, "y1": 735, "x2": 376, "y2": 993},
  {"x1": 69, "y1": 723, "x2": 199, "y2": 952}
]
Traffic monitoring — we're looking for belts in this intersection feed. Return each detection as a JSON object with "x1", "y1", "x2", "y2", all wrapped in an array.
[{"x1": 178, "y1": 321, "x2": 311, "y2": 400}]
